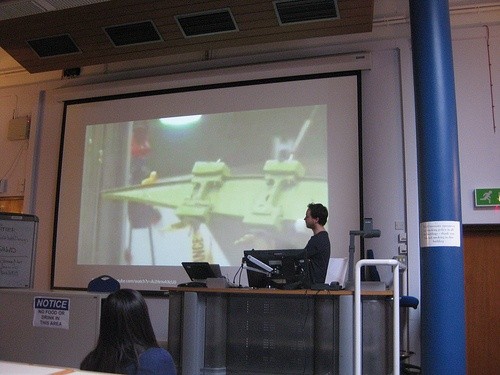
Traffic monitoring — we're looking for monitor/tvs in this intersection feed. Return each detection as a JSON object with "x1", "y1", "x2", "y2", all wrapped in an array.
[{"x1": 243, "y1": 249, "x2": 307, "y2": 287}]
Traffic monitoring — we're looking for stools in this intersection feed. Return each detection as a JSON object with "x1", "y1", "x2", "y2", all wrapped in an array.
[{"x1": 365, "y1": 249, "x2": 421, "y2": 375}]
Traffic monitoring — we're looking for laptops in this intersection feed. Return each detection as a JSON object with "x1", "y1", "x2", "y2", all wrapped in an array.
[{"x1": 181, "y1": 261, "x2": 217, "y2": 283}]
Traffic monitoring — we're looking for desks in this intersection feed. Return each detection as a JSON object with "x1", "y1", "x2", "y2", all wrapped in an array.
[{"x1": 159, "y1": 286, "x2": 394, "y2": 375}]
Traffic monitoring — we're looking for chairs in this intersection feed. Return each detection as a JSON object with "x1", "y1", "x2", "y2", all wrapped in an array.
[{"x1": 88, "y1": 275, "x2": 120, "y2": 292}]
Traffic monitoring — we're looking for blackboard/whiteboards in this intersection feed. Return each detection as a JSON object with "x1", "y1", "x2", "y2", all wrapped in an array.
[{"x1": 0, "y1": 214, "x2": 39, "y2": 289}]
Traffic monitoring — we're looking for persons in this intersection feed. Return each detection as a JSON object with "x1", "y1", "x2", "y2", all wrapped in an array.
[
  {"x1": 80, "y1": 289, "x2": 176, "y2": 375},
  {"x1": 304, "y1": 204, "x2": 331, "y2": 285}
]
